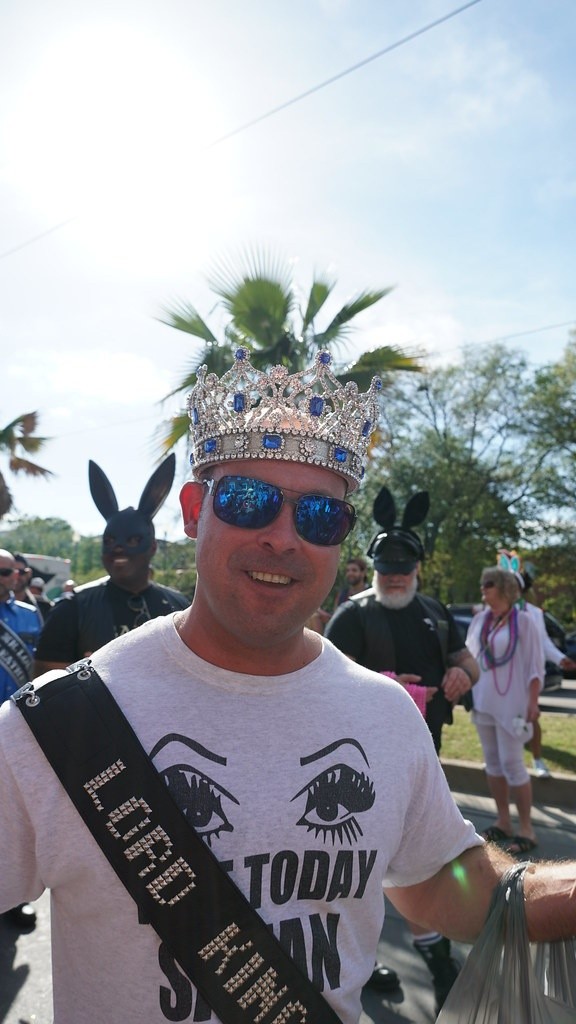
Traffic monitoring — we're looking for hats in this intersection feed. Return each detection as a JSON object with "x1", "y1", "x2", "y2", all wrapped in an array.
[
  {"x1": 30, "y1": 577, "x2": 45, "y2": 588},
  {"x1": 370, "y1": 531, "x2": 421, "y2": 575}
]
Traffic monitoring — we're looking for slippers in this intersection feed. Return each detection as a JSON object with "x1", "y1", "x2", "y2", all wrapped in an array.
[
  {"x1": 480, "y1": 826, "x2": 510, "y2": 842},
  {"x1": 511, "y1": 834, "x2": 538, "y2": 854}
]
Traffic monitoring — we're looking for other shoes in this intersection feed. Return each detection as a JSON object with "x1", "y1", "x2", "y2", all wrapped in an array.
[{"x1": 533, "y1": 759, "x2": 549, "y2": 777}]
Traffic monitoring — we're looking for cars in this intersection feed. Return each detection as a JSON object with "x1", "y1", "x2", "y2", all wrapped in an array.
[{"x1": 440, "y1": 601, "x2": 576, "y2": 679}]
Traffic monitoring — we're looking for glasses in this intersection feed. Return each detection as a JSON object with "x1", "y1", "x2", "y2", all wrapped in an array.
[
  {"x1": 199, "y1": 474, "x2": 359, "y2": 546},
  {"x1": 0, "y1": 568, "x2": 17, "y2": 577},
  {"x1": 18, "y1": 569, "x2": 29, "y2": 576},
  {"x1": 480, "y1": 580, "x2": 500, "y2": 588}
]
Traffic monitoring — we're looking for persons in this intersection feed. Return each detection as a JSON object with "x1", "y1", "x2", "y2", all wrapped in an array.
[
  {"x1": 497, "y1": 554, "x2": 574, "y2": 777},
  {"x1": 0, "y1": 452, "x2": 187, "y2": 923},
  {"x1": 325, "y1": 485, "x2": 480, "y2": 757},
  {"x1": 465, "y1": 566, "x2": 545, "y2": 851},
  {"x1": 0, "y1": 346, "x2": 576, "y2": 1024},
  {"x1": 305, "y1": 560, "x2": 367, "y2": 637}
]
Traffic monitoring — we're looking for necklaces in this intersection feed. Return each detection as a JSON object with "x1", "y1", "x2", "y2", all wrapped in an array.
[
  {"x1": 477, "y1": 606, "x2": 517, "y2": 695},
  {"x1": 380, "y1": 671, "x2": 428, "y2": 719}
]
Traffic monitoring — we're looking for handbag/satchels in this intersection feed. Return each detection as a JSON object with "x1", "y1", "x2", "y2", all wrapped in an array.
[{"x1": 434, "y1": 859, "x2": 576, "y2": 1024}]
